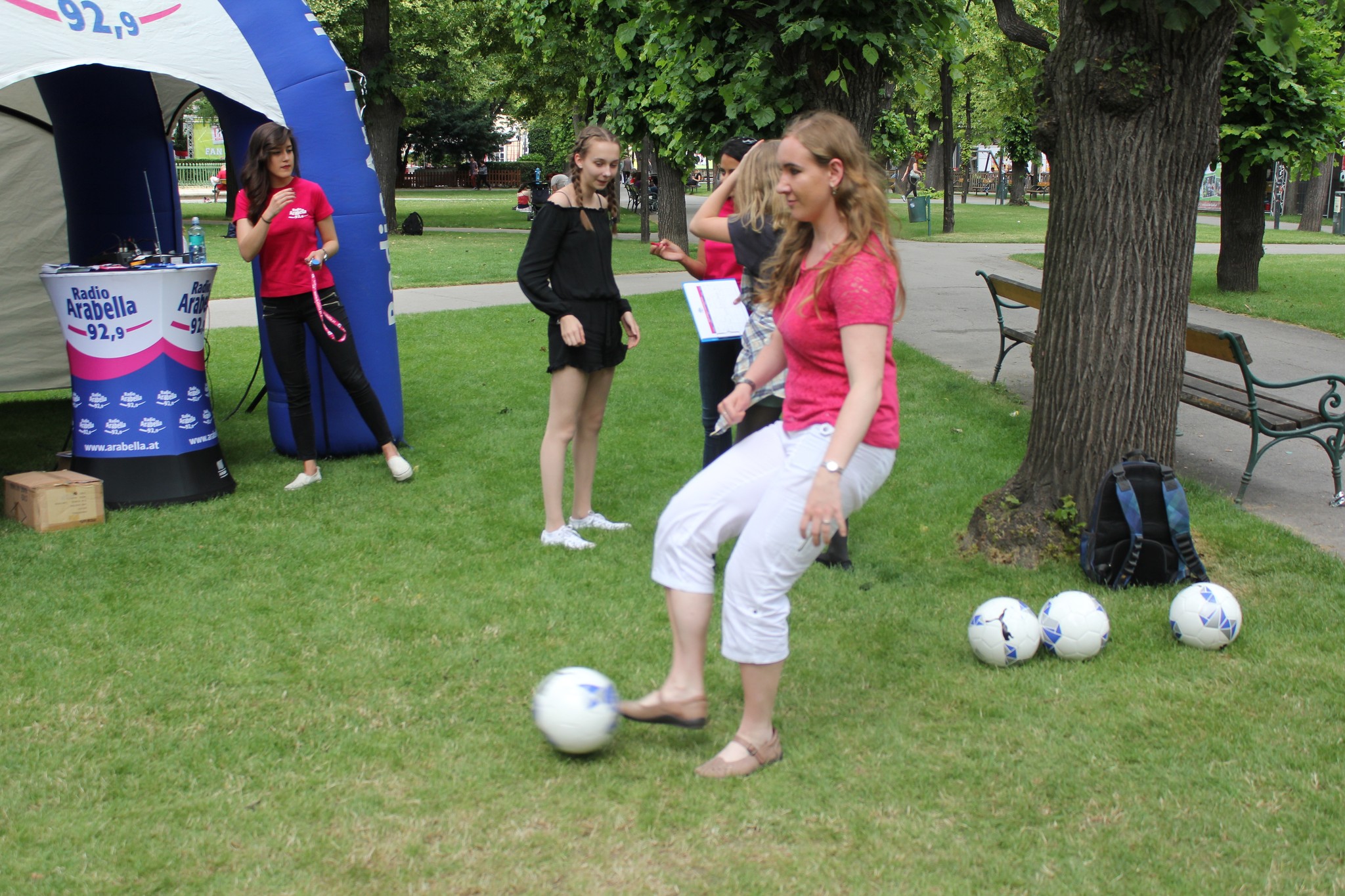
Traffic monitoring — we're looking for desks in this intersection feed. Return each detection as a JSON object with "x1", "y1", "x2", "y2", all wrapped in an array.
[{"x1": 38, "y1": 264, "x2": 237, "y2": 510}]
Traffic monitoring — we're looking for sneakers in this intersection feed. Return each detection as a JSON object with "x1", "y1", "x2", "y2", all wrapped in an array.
[
  {"x1": 902, "y1": 195, "x2": 906, "y2": 202},
  {"x1": 540, "y1": 526, "x2": 596, "y2": 551},
  {"x1": 568, "y1": 514, "x2": 632, "y2": 531},
  {"x1": 284, "y1": 466, "x2": 322, "y2": 490},
  {"x1": 385, "y1": 451, "x2": 413, "y2": 481}
]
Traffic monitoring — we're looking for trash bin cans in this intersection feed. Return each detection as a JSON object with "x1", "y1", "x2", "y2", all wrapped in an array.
[
  {"x1": 1333, "y1": 190, "x2": 1345, "y2": 236},
  {"x1": 996, "y1": 183, "x2": 1007, "y2": 199},
  {"x1": 906, "y1": 196, "x2": 927, "y2": 223}
]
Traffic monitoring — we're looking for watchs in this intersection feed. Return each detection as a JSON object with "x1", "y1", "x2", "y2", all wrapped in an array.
[
  {"x1": 818, "y1": 461, "x2": 846, "y2": 476},
  {"x1": 321, "y1": 249, "x2": 328, "y2": 263}
]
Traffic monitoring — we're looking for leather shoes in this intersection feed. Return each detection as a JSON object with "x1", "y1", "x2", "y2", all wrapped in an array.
[
  {"x1": 618, "y1": 689, "x2": 707, "y2": 730},
  {"x1": 693, "y1": 726, "x2": 782, "y2": 780}
]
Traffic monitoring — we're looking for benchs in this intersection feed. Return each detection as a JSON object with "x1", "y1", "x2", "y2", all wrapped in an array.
[
  {"x1": 1027, "y1": 182, "x2": 1050, "y2": 200},
  {"x1": 975, "y1": 269, "x2": 1345, "y2": 510},
  {"x1": 953, "y1": 178, "x2": 970, "y2": 194},
  {"x1": 887, "y1": 178, "x2": 896, "y2": 193},
  {"x1": 622, "y1": 183, "x2": 658, "y2": 213},
  {"x1": 212, "y1": 179, "x2": 227, "y2": 203},
  {"x1": 685, "y1": 180, "x2": 705, "y2": 193},
  {"x1": 972, "y1": 179, "x2": 995, "y2": 196}
]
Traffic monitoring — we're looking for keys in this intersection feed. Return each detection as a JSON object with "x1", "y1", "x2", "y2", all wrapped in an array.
[{"x1": 309, "y1": 256, "x2": 314, "y2": 267}]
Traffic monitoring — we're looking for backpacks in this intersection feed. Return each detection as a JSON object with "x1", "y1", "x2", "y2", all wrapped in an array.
[{"x1": 1078, "y1": 449, "x2": 1210, "y2": 583}]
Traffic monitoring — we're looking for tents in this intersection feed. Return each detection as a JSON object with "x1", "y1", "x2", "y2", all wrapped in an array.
[{"x1": 1, "y1": 0, "x2": 405, "y2": 458}]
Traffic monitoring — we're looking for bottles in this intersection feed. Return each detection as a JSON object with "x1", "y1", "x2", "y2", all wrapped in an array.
[{"x1": 188, "y1": 215, "x2": 206, "y2": 264}]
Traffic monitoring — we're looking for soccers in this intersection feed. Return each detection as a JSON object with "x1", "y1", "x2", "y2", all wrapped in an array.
[
  {"x1": 1039, "y1": 590, "x2": 1109, "y2": 662},
  {"x1": 534, "y1": 667, "x2": 620, "y2": 754},
  {"x1": 968, "y1": 596, "x2": 1041, "y2": 667},
  {"x1": 1170, "y1": 583, "x2": 1243, "y2": 650}
]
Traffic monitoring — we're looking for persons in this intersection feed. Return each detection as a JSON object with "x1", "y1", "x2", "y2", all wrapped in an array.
[
  {"x1": 891, "y1": 169, "x2": 899, "y2": 193},
  {"x1": 233, "y1": 122, "x2": 414, "y2": 490},
  {"x1": 474, "y1": 159, "x2": 492, "y2": 191},
  {"x1": 620, "y1": 114, "x2": 909, "y2": 779},
  {"x1": 209, "y1": 165, "x2": 227, "y2": 203},
  {"x1": 517, "y1": 124, "x2": 640, "y2": 548},
  {"x1": 901, "y1": 156, "x2": 922, "y2": 200},
  {"x1": 690, "y1": 137, "x2": 852, "y2": 567},
  {"x1": 988, "y1": 162, "x2": 1044, "y2": 195},
  {"x1": 469, "y1": 157, "x2": 479, "y2": 189},
  {"x1": 546, "y1": 171, "x2": 571, "y2": 195},
  {"x1": 517, "y1": 183, "x2": 532, "y2": 212},
  {"x1": 650, "y1": 138, "x2": 756, "y2": 472},
  {"x1": 619, "y1": 153, "x2": 702, "y2": 214}
]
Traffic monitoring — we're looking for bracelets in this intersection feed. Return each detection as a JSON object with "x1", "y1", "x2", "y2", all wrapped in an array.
[
  {"x1": 736, "y1": 377, "x2": 757, "y2": 392},
  {"x1": 260, "y1": 215, "x2": 272, "y2": 224}
]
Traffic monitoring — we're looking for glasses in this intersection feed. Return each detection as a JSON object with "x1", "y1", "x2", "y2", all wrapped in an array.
[{"x1": 729, "y1": 136, "x2": 757, "y2": 145}]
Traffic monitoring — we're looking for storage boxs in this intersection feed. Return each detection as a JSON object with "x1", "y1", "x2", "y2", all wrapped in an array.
[{"x1": 3, "y1": 469, "x2": 105, "y2": 533}]
